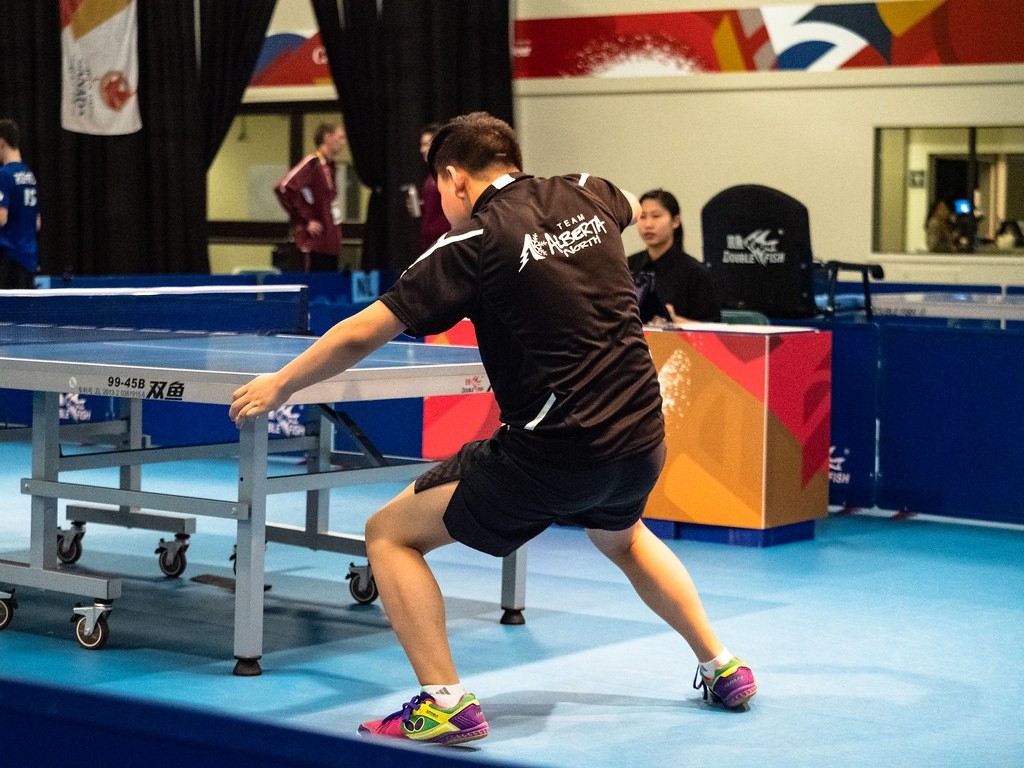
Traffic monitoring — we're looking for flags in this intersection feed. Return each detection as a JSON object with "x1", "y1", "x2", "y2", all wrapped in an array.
[{"x1": 58, "y1": 0, "x2": 144, "y2": 136}]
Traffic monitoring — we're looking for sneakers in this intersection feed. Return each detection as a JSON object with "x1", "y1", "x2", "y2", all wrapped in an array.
[
  {"x1": 693, "y1": 657, "x2": 757, "y2": 707},
  {"x1": 356, "y1": 691, "x2": 489, "y2": 746}
]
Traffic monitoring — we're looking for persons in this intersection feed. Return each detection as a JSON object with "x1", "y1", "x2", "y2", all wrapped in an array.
[
  {"x1": 272, "y1": 121, "x2": 348, "y2": 274},
  {"x1": 925, "y1": 197, "x2": 959, "y2": 254},
  {"x1": 0, "y1": 119, "x2": 42, "y2": 290},
  {"x1": 227, "y1": 111, "x2": 757, "y2": 748},
  {"x1": 627, "y1": 186, "x2": 723, "y2": 328},
  {"x1": 405, "y1": 122, "x2": 453, "y2": 252}
]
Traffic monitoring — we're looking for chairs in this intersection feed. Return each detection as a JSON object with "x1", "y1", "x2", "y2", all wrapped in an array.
[{"x1": 700, "y1": 185, "x2": 887, "y2": 318}]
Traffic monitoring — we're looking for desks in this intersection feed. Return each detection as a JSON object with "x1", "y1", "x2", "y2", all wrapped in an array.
[
  {"x1": 872, "y1": 290, "x2": 1024, "y2": 330},
  {"x1": 421, "y1": 314, "x2": 834, "y2": 548},
  {"x1": 0, "y1": 324, "x2": 529, "y2": 676}
]
